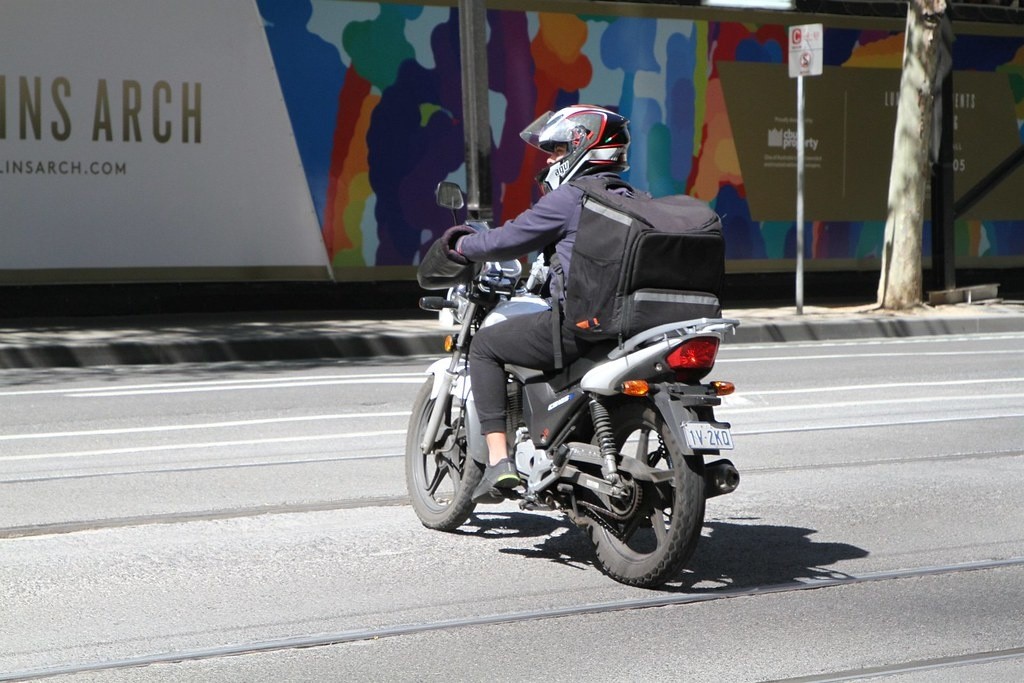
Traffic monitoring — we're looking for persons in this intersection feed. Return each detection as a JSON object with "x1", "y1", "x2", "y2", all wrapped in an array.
[{"x1": 455, "y1": 104, "x2": 631, "y2": 504}]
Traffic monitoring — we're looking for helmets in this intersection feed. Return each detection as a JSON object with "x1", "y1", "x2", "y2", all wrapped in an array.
[{"x1": 534, "y1": 104, "x2": 630, "y2": 199}]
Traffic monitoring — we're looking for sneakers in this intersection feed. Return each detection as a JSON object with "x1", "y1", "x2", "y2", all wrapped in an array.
[{"x1": 471, "y1": 458, "x2": 520, "y2": 503}]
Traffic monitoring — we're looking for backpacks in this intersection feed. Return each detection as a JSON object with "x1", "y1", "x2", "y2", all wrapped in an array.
[{"x1": 547, "y1": 179, "x2": 725, "y2": 370}]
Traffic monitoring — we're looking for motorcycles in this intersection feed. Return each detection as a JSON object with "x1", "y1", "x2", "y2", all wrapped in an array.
[{"x1": 404, "y1": 182, "x2": 742, "y2": 589}]
